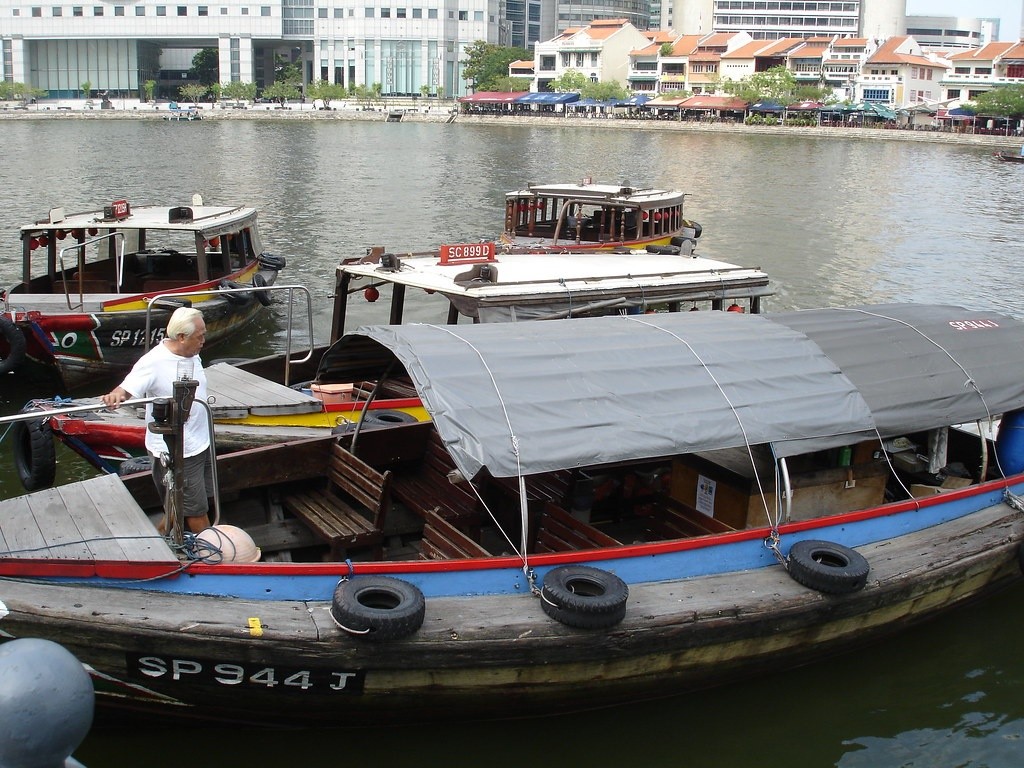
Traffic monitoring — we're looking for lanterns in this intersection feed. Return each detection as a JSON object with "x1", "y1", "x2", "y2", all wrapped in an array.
[
  {"x1": 517, "y1": 202, "x2": 544, "y2": 212},
  {"x1": 365, "y1": 287, "x2": 379, "y2": 303},
  {"x1": 643, "y1": 211, "x2": 679, "y2": 221},
  {"x1": 727, "y1": 304, "x2": 744, "y2": 314},
  {"x1": 424, "y1": 289, "x2": 435, "y2": 295},
  {"x1": 30, "y1": 228, "x2": 98, "y2": 251},
  {"x1": 209, "y1": 228, "x2": 249, "y2": 247},
  {"x1": 640, "y1": 308, "x2": 700, "y2": 314}
]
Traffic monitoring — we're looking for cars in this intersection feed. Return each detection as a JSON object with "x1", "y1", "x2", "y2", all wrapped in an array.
[{"x1": 992, "y1": 150, "x2": 1024, "y2": 162}]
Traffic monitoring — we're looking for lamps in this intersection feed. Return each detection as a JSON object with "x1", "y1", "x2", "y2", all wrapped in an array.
[{"x1": 160, "y1": 452, "x2": 172, "y2": 469}]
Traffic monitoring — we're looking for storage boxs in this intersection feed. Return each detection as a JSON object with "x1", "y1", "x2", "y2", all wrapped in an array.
[
  {"x1": 893, "y1": 453, "x2": 927, "y2": 472},
  {"x1": 910, "y1": 476, "x2": 973, "y2": 499},
  {"x1": 310, "y1": 383, "x2": 353, "y2": 403}
]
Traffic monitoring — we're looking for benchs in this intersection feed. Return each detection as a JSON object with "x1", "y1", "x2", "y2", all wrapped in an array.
[
  {"x1": 516, "y1": 222, "x2": 636, "y2": 241},
  {"x1": 189, "y1": 106, "x2": 203, "y2": 109},
  {"x1": 274, "y1": 107, "x2": 285, "y2": 110},
  {"x1": 144, "y1": 280, "x2": 199, "y2": 294},
  {"x1": 370, "y1": 376, "x2": 418, "y2": 400},
  {"x1": 632, "y1": 496, "x2": 738, "y2": 542},
  {"x1": 233, "y1": 106, "x2": 243, "y2": 109},
  {"x1": 14, "y1": 107, "x2": 28, "y2": 110},
  {"x1": 53, "y1": 280, "x2": 109, "y2": 294},
  {"x1": 319, "y1": 107, "x2": 331, "y2": 110},
  {"x1": 487, "y1": 468, "x2": 579, "y2": 554},
  {"x1": 362, "y1": 108, "x2": 374, "y2": 111},
  {"x1": 392, "y1": 428, "x2": 486, "y2": 544},
  {"x1": 351, "y1": 380, "x2": 378, "y2": 402},
  {"x1": 419, "y1": 506, "x2": 511, "y2": 560},
  {"x1": 408, "y1": 109, "x2": 418, "y2": 112},
  {"x1": 72, "y1": 271, "x2": 116, "y2": 292},
  {"x1": 535, "y1": 502, "x2": 644, "y2": 554},
  {"x1": 284, "y1": 436, "x2": 394, "y2": 562},
  {"x1": 58, "y1": 107, "x2": 71, "y2": 110}
]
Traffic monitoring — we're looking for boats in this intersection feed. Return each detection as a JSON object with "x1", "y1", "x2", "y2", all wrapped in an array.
[
  {"x1": 493, "y1": 174, "x2": 698, "y2": 252},
  {"x1": 0, "y1": 194, "x2": 286, "y2": 379},
  {"x1": 0, "y1": 301, "x2": 1023, "y2": 717},
  {"x1": 26, "y1": 242, "x2": 775, "y2": 478}
]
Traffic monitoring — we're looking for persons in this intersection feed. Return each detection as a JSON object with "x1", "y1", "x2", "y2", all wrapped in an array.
[{"x1": 100, "y1": 307, "x2": 212, "y2": 535}]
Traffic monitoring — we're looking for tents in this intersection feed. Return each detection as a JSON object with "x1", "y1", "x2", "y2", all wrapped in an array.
[{"x1": 459, "y1": 91, "x2": 1024, "y2": 136}]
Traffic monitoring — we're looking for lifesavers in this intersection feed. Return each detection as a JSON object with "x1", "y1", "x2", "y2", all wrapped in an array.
[
  {"x1": 11, "y1": 407, "x2": 57, "y2": 492},
  {"x1": 257, "y1": 250, "x2": 288, "y2": 271},
  {"x1": 0, "y1": 315, "x2": 26, "y2": 372},
  {"x1": 120, "y1": 453, "x2": 158, "y2": 475},
  {"x1": 332, "y1": 421, "x2": 386, "y2": 435},
  {"x1": 253, "y1": 271, "x2": 275, "y2": 303},
  {"x1": 782, "y1": 535, "x2": 869, "y2": 591},
  {"x1": 328, "y1": 571, "x2": 431, "y2": 641},
  {"x1": 219, "y1": 277, "x2": 250, "y2": 305},
  {"x1": 535, "y1": 564, "x2": 633, "y2": 630},
  {"x1": 155, "y1": 296, "x2": 198, "y2": 309},
  {"x1": 369, "y1": 407, "x2": 422, "y2": 432}
]
